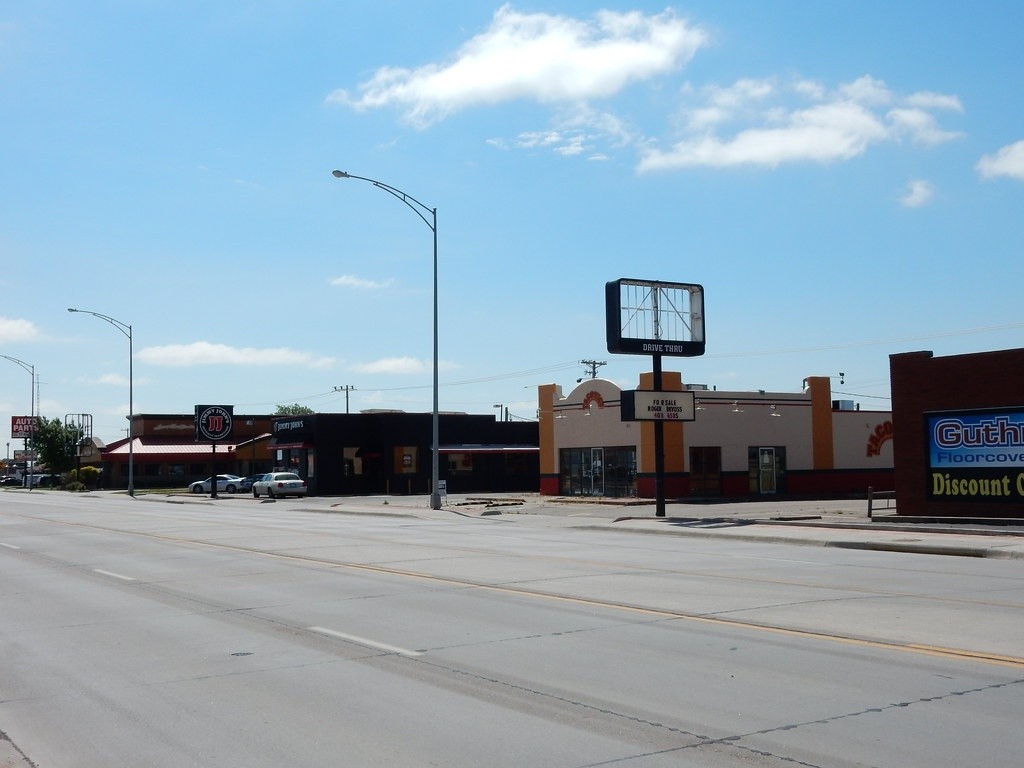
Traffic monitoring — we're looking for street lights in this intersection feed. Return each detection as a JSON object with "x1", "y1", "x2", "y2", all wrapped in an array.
[
  {"x1": 332, "y1": 169, "x2": 442, "y2": 510},
  {"x1": 68, "y1": 308, "x2": 134, "y2": 497}
]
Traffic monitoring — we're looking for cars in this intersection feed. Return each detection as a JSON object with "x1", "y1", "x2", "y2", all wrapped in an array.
[
  {"x1": 251, "y1": 472, "x2": 307, "y2": 499},
  {"x1": 0, "y1": 476, "x2": 23, "y2": 485}
]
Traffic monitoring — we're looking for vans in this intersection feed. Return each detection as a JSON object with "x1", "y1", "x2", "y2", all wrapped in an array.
[{"x1": 37, "y1": 476, "x2": 60, "y2": 487}]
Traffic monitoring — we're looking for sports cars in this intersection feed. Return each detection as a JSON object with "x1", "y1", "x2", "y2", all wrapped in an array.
[{"x1": 189, "y1": 474, "x2": 252, "y2": 494}]
